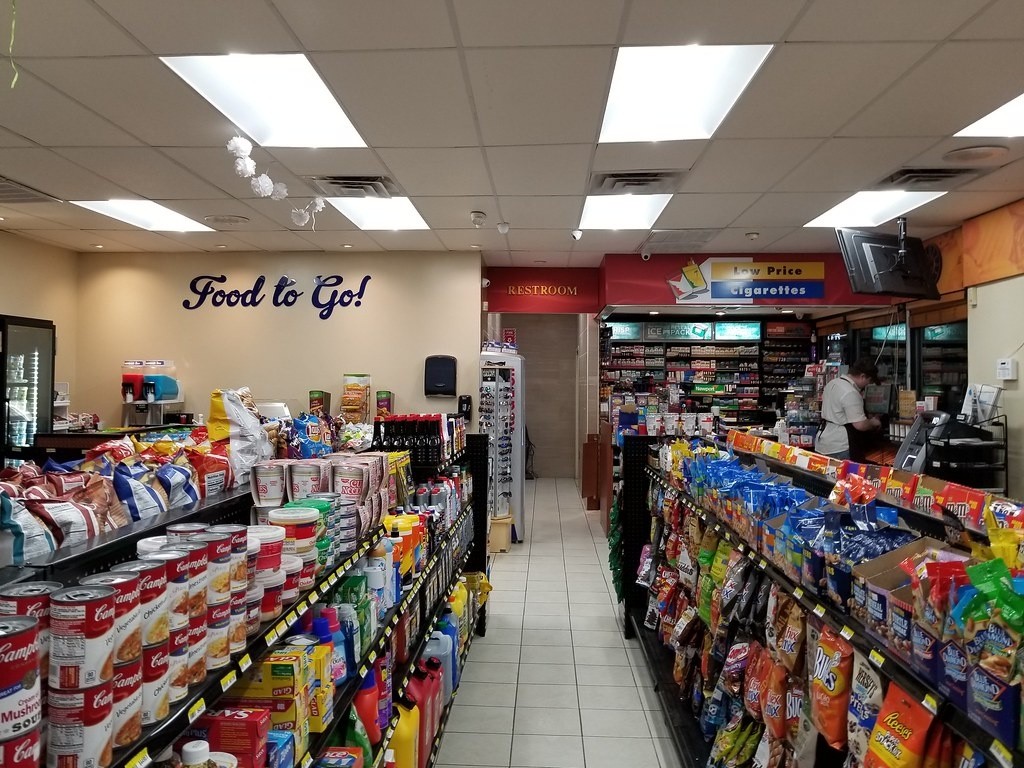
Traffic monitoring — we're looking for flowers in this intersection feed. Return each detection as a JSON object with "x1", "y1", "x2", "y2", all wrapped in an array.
[{"x1": 227, "y1": 136, "x2": 325, "y2": 227}]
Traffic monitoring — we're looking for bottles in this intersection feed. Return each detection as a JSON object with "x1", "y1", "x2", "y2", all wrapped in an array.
[
  {"x1": 283, "y1": 413, "x2": 472, "y2": 746},
  {"x1": 181, "y1": 740, "x2": 218, "y2": 768}
]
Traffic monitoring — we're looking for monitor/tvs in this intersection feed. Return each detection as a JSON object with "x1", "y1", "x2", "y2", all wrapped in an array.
[
  {"x1": 863, "y1": 384, "x2": 895, "y2": 416},
  {"x1": 834, "y1": 227, "x2": 940, "y2": 300}
]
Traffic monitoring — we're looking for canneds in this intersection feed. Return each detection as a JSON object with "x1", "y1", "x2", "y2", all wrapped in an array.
[{"x1": 0, "y1": 455, "x2": 364, "y2": 768}]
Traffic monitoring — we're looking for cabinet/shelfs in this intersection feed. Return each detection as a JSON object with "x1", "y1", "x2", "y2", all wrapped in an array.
[
  {"x1": 620, "y1": 434, "x2": 1024, "y2": 768},
  {"x1": 759, "y1": 339, "x2": 812, "y2": 409},
  {"x1": 925, "y1": 415, "x2": 1009, "y2": 498},
  {"x1": 0, "y1": 433, "x2": 490, "y2": 768},
  {"x1": 665, "y1": 348, "x2": 761, "y2": 413},
  {"x1": 340, "y1": 374, "x2": 371, "y2": 425},
  {"x1": 52, "y1": 382, "x2": 69, "y2": 431},
  {"x1": 601, "y1": 343, "x2": 666, "y2": 384},
  {"x1": 871, "y1": 339, "x2": 968, "y2": 388}
]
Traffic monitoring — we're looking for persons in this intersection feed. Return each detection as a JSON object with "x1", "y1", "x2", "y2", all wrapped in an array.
[{"x1": 815, "y1": 361, "x2": 881, "y2": 461}]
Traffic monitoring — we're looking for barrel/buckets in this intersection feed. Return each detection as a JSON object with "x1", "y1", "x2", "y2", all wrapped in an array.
[{"x1": 387, "y1": 577, "x2": 471, "y2": 768}]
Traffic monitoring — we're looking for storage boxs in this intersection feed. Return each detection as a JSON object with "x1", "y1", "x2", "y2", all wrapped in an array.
[
  {"x1": 268, "y1": 644, "x2": 331, "y2": 695},
  {"x1": 602, "y1": 344, "x2": 759, "y2": 411},
  {"x1": 265, "y1": 730, "x2": 279, "y2": 768},
  {"x1": 227, "y1": 745, "x2": 267, "y2": 768},
  {"x1": 267, "y1": 730, "x2": 294, "y2": 768},
  {"x1": 223, "y1": 649, "x2": 308, "y2": 698},
  {"x1": 292, "y1": 719, "x2": 309, "y2": 767},
  {"x1": 215, "y1": 683, "x2": 311, "y2": 732},
  {"x1": 316, "y1": 747, "x2": 364, "y2": 768},
  {"x1": 647, "y1": 443, "x2": 974, "y2": 660},
  {"x1": 306, "y1": 683, "x2": 335, "y2": 733},
  {"x1": 174, "y1": 707, "x2": 271, "y2": 753}
]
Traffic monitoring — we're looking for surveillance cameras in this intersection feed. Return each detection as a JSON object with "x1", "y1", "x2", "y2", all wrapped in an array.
[
  {"x1": 482, "y1": 278, "x2": 490, "y2": 287},
  {"x1": 497, "y1": 223, "x2": 509, "y2": 234},
  {"x1": 641, "y1": 251, "x2": 651, "y2": 261},
  {"x1": 571, "y1": 230, "x2": 583, "y2": 240}
]
